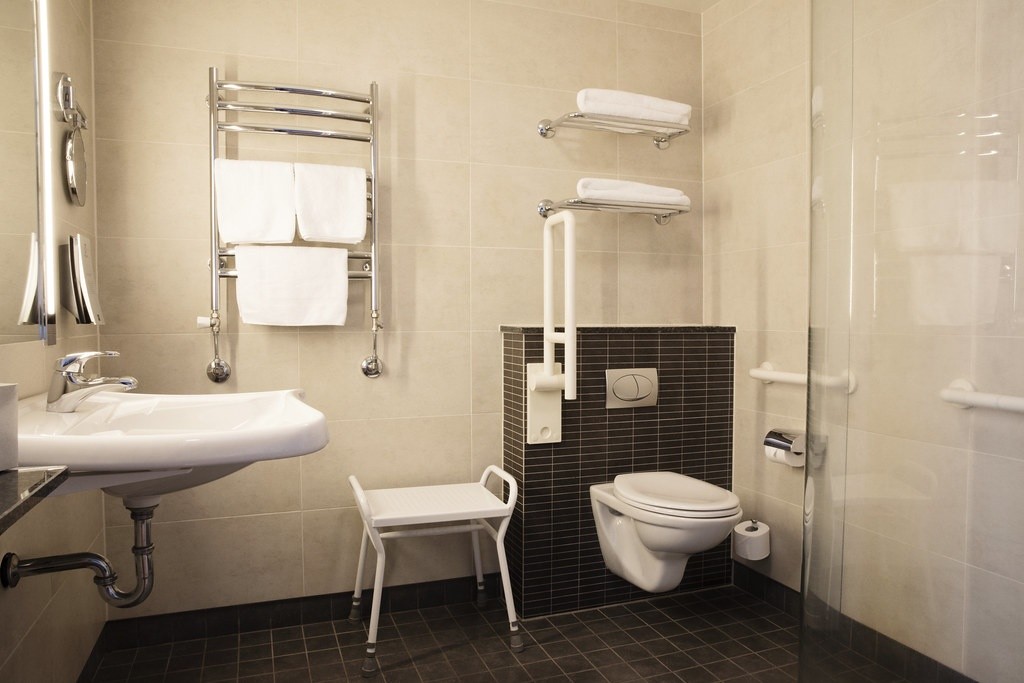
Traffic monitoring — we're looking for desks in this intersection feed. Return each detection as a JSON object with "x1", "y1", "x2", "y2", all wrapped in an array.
[{"x1": 347, "y1": 465, "x2": 525, "y2": 678}]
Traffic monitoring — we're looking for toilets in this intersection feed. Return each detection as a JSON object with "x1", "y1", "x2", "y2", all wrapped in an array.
[{"x1": 589, "y1": 470, "x2": 742, "y2": 594}]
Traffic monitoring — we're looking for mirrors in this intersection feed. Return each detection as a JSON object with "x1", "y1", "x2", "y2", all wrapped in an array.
[{"x1": 1, "y1": 0, "x2": 45, "y2": 346}]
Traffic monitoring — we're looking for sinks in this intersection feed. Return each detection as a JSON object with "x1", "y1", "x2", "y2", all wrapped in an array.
[{"x1": 19, "y1": 381, "x2": 329, "y2": 508}]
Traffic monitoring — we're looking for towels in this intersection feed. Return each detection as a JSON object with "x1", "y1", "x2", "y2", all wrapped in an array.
[
  {"x1": 577, "y1": 178, "x2": 690, "y2": 205},
  {"x1": 213, "y1": 158, "x2": 295, "y2": 245},
  {"x1": 577, "y1": 86, "x2": 690, "y2": 134},
  {"x1": 293, "y1": 162, "x2": 367, "y2": 245},
  {"x1": 234, "y1": 247, "x2": 349, "y2": 326}
]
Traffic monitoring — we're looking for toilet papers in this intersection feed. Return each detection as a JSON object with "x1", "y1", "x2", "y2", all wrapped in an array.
[
  {"x1": 765, "y1": 442, "x2": 806, "y2": 469},
  {"x1": 729, "y1": 519, "x2": 771, "y2": 561}
]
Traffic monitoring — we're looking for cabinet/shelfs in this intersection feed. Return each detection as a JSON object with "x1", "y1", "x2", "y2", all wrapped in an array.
[{"x1": 537, "y1": 113, "x2": 691, "y2": 225}]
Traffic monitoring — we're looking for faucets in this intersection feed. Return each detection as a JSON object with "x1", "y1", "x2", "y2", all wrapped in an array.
[{"x1": 47, "y1": 348, "x2": 138, "y2": 412}]
